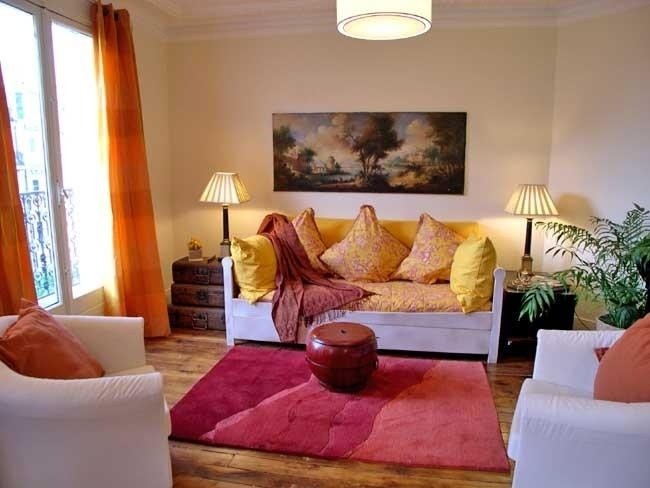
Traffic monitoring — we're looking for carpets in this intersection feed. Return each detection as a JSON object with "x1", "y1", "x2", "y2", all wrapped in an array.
[{"x1": 167, "y1": 346, "x2": 509, "y2": 472}]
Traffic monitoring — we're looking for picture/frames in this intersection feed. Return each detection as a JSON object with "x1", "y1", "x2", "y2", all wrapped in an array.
[{"x1": 272, "y1": 113, "x2": 465, "y2": 195}]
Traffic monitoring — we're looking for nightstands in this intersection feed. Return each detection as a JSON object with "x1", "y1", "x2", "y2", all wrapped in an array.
[
  {"x1": 504, "y1": 271, "x2": 578, "y2": 358},
  {"x1": 170, "y1": 258, "x2": 226, "y2": 331}
]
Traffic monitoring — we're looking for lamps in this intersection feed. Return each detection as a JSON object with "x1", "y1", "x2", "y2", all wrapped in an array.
[
  {"x1": 505, "y1": 186, "x2": 557, "y2": 289},
  {"x1": 336, "y1": 0, "x2": 431, "y2": 41},
  {"x1": 197, "y1": 172, "x2": 250, "y2": 257}
]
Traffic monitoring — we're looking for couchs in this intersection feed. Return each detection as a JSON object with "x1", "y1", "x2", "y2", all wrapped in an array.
[
  {"x1": 510, "y1": 327, "x2": 650, "y2": 488},
  {"x1": 0, "y1": 315, "x2": 173, "y2": 488},
  {"x1": 222, "y1": 218, "x2": 504, "y2": 364}
]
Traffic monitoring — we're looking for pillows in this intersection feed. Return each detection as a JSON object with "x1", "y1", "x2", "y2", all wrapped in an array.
[
  {"x1": 450, "y1": 234, "x2": 496, "y2": 315},
  {"x1": 230, "y1": 209, "x2": 328, "y2": 301},
  {"x1": 593, "y1": 314, "x2": 650, "y2": 402},
  {"x1": 291, "y1": 210, "x2": 331, "y2": 275},
  {"x1": 319, "y1": 205, "x2": 409, "y2": 282},
  {"x1": 0, "y1": 308, "x2": 106, "y2": 381},
  {"x1": 394, "y1": 214, "x2": 466, "y2": 285}
]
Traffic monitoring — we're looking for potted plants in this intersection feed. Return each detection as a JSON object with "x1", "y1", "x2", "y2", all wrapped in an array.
[
  {"x1": 187, "y1": 240, "x2": 201, "y2": 261},
  {"x1": 516, "y1": 207, "x2": 650, "y2": 330}
]
